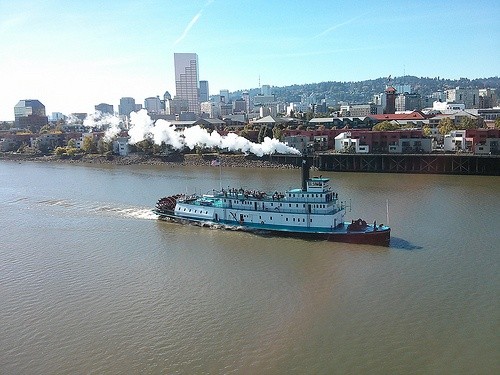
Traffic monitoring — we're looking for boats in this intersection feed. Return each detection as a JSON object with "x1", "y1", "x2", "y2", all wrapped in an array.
[{"x1": 151, "y1": 158, "x2": 391, "y2": 248}]
